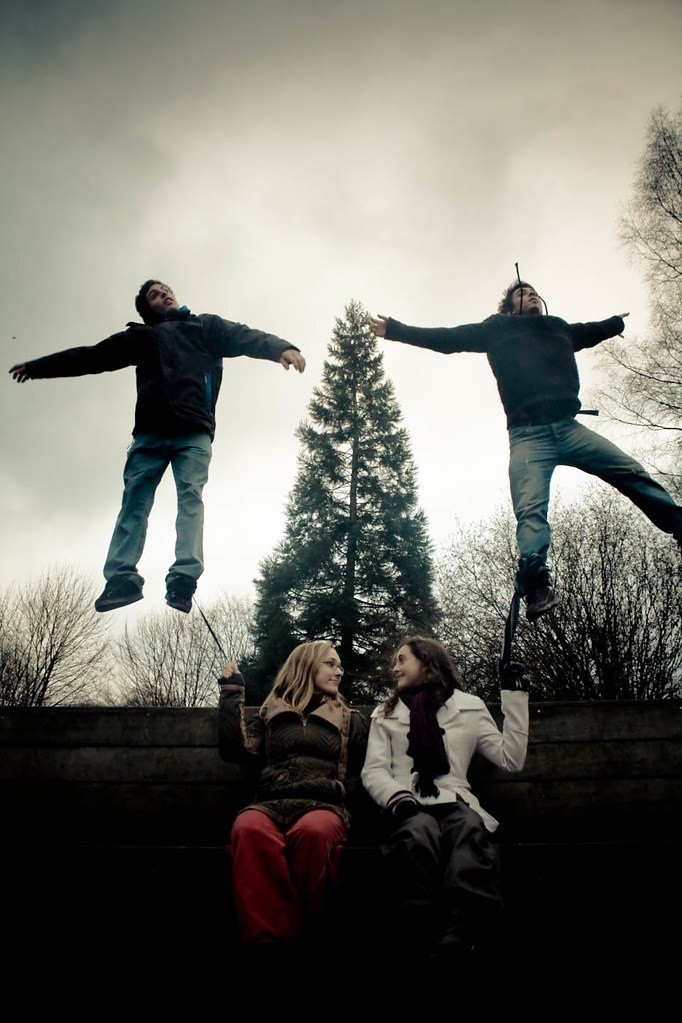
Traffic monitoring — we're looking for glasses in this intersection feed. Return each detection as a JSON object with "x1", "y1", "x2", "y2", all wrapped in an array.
[{"x1": 321, "y1": 660, "x2": 344, "y2": 675}]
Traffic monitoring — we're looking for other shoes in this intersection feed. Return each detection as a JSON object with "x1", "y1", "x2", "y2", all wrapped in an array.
[
  {"x1": 95, "y1": 581, "x2": 144, "y2": 613},
  {"x1": 165, "y1": 584, "x2": 194, "y2": 613},
  {"x1": 526, "y1": 585, "x2": 559, "y2": 619}
]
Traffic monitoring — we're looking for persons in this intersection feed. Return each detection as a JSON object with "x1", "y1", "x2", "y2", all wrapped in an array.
[
  {"x1": 367, "y1": 263, "x2": 682, "y2": 620},
  {"x1": 216, "y1": 639, "x2": 369, "y2": 945},
  {"x1": 360, "y1": 635, "x2": 530, "y2": 951},
  {"x1": 9, "y1": 279, "x2": 305, "y2": 613}
]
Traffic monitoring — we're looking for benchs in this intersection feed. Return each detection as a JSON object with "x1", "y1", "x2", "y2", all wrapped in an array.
[{"x1": 0, "y1": 701, "x2": 682, "y2": 928}]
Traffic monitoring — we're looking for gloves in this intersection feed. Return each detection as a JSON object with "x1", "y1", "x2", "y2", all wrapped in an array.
[
  {"x1": 314, "y1": 778, "x2": 347, "y2": 803},
  {"x1": 506, "y1": 663, "x2": 530, "y2": 692},
  {"x1": 395, "y1": 800, "x2": 427, "y2": 818}
]
